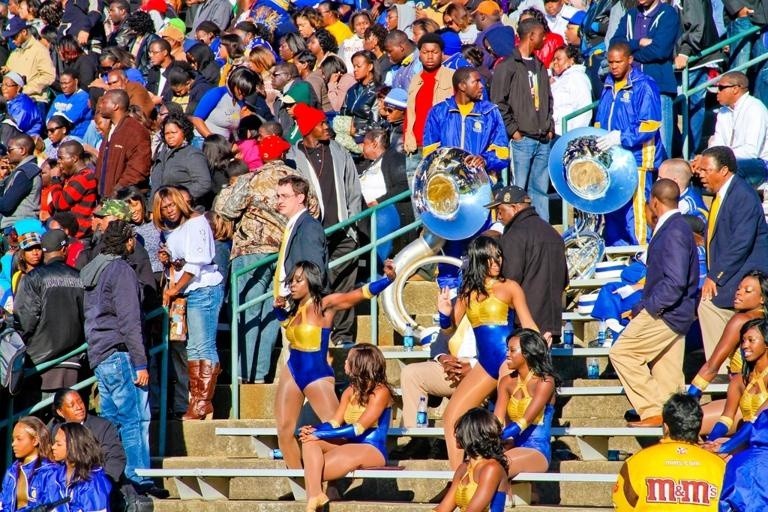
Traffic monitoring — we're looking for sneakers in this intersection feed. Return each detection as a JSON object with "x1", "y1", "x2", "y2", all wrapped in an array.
[{"x1": 133, "y1": 481, "x2": 170, "y2": 499}]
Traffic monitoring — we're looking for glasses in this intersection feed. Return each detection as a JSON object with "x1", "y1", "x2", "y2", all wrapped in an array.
[
  {"x1": 275, "y1": 193, "x2": 303, "y2": 201},
  {"x1": 718, "y1": 84, "x2": 741, "y2": 89},
  {"x1": 47, "y1": 126, "x2": 67, "y2": 132},
  {"x1": 108, "y1": 81, "x2": 116, "y2": 85},
  {"x1": 273, "y1": 71, "x2": 292, "y2": 77},
  {"x1": 697, "y1": 167, "x2": 726, "y2": 176},
  {"x1": 0, "y1": 83, "x2": 16, "y2": 88},
  {"x1": 7, "y1": 146, "x2": 27, "y2": 152},
  {"x1": 99, "y1": 61, "x2": 116, "y2": 71},
  {"x1": 384, "y1": 106, "x2": 400, "y2": 112},
  {"x1": 148, "y1": 51, "x2": 160, "y2": 57}
]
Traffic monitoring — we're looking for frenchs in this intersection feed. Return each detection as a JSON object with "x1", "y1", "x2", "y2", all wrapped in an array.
[
  {"x1": 378, "y1": 147, "x2": 495, "y2": 343},
  {"x1": 549, "y1": 127, "x2": 639, "y2": 312}
]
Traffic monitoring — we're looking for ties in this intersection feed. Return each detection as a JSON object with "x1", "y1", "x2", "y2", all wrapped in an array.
[{"x1": 274, "y1": 221, "x2": 291, "y2": 298}]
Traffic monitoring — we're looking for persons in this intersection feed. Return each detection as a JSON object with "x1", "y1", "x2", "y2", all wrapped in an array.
[
  {"x1": 83, "y1": 219, "x2": 170, "y2": 500},
  {"x1": 1, "y1": 1, "x2": 768, "y2": 478},
  {"x1": 48, "y1": 386, "x2": 127, "y2": 485},
  {"x1": 717, "y1": 406, "x2": 767, "y2": 511},
  {"x1": 23, "y1": 420, "x2": 113, "y2": 511},
  {"x1": 611, "y1": 392, "x2": 729, "y2": 511},
  {"x1": 431, "y1": 406, "x2": 509, "y2": 511},
  {"x1": 0, "y1": 414, "x2": 55, "y2": 512},
  {"x1": 295, "y1": 341, "x2": 397, "y2": 511}
]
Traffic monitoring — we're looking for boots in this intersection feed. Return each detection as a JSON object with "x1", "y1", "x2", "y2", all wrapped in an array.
[
  {"x1": 183, "y1": 361, "x2": 200, "y2": 415},
  {"x1": 182, "y1": 361, "x2": 221, "y2": 422}
]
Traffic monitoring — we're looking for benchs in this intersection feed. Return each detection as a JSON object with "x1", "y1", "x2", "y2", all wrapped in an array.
[{"x1": 135, "y1": 466, "x2": 620, "y2": 504}]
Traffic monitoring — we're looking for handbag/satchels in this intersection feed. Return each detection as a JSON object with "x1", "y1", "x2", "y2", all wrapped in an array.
[{"x1": 156, "y1": 264, "x2": 187, "y2": 342}]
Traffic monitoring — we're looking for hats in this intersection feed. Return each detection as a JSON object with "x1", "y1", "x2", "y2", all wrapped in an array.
[
  {"x1": 161, "y1": 25, "x2": 183, "y2": 41},
  {"x1": 260, "y1": 135, "x2": 289, "y2": 160},
  {"x1": 484, "y1": 185, "x2": 531, "y2": 209},
  {"x1": 142, "y1": 1, "x2": 169, "y2": 12},
  {"x1": 385, "y1": 88, "x2": 409, "y2": 109},
  {"x1": 471, "y1": 0, "x2": 504, "y2": 18},
  {"x1": 18, "y1": 199, "x2": 132, "y2": 251},
  {"x1": 563, "y1": 9, "x2": 587, "y2": 27},
  {"x1": 293, "y1": 103, "x2": 324, "y2": 135},
  {"x1": 3, "y1": 17, "x2": 27, "y2": 38}
]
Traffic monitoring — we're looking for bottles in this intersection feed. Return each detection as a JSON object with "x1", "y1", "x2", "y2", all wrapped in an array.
[
  {"x1": 402, "y1": 322, "x2": 414, "y2": 350},
  {"x1": 606, "y1": 448, "x2": 632, "y2": 461},
  {"x1": 415, "y1": 397, "x2": 428, "y2": 428},
  {"x1": 597, "y1": 318, "x2": 606, "y2": 347},
  {"x1": 587, "y1": 358, "x2": 598, "y2": 379},
  {"x1": 563, "y1": 319, "x2": 573, "y2": 348}
]
